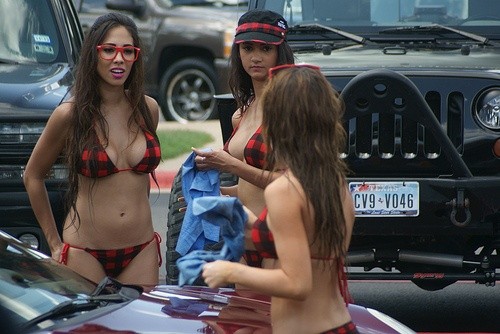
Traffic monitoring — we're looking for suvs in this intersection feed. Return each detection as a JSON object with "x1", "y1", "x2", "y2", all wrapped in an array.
[
  {"x1": 2, "y1": 1, "x2": 98, "y2": 246},
  {"x1": 238, "y1": 0, "x2": 499, "y2": 290}
]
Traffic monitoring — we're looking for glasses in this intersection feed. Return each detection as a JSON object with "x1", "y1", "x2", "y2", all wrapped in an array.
[
  {"x1": 267, "y1": 63, "x2": 321, "y2": 80},
  {"x1": 96, "y1": 44, "x2": 141, "y2": 61}
]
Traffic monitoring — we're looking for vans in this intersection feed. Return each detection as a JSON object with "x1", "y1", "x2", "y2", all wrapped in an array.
[{"x1": 76, "y1": 0, "x2": 233, "y2": 121}]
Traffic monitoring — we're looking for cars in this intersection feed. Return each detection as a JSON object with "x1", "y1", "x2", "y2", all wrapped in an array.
[{"x1": 2, "y1": 234, "x2": 414, "y2": 334}]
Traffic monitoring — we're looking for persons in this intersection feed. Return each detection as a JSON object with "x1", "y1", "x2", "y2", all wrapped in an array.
[
  {"x1": 191, "y1": 8, "x2": 295, "y2": 289},
  {"x1": 23, "y1": 12, "x2": 164, "y2": 286},
  {"x1": 203, "y1": 62, "x2": 361, "y2": 333}
]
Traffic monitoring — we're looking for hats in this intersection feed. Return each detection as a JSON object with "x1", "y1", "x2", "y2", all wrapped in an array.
[{"x1": 233, "y1": 8, "x2": 288, "y2": 45}]
